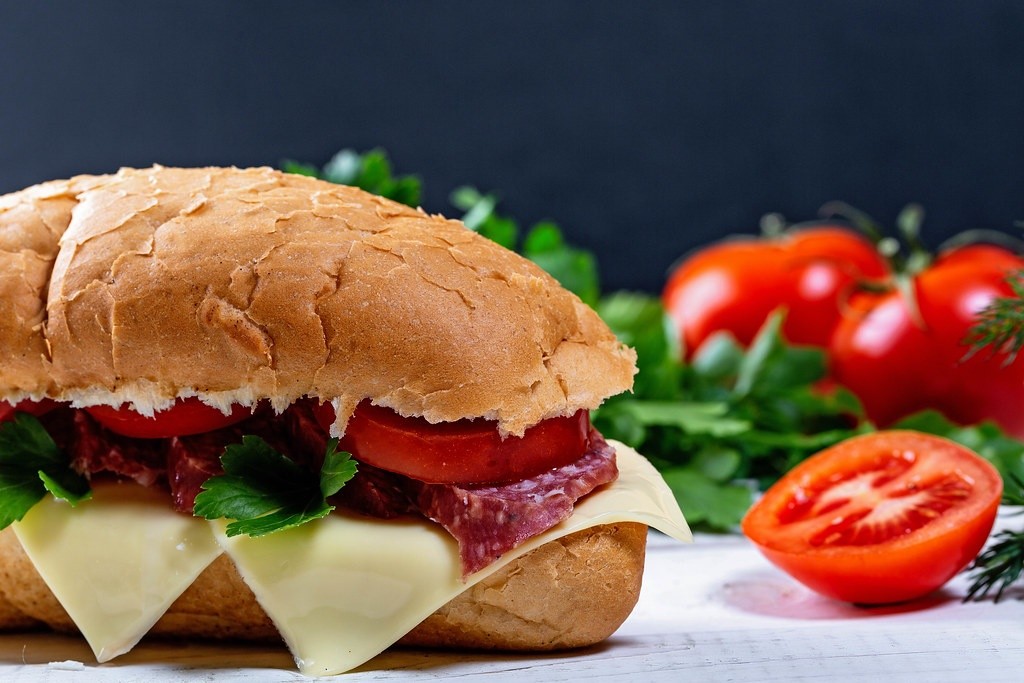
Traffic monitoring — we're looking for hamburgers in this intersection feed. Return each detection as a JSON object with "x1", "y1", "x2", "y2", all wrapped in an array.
[{"x1": 0, "y1": 166, "x2": 692, "y2": 676}]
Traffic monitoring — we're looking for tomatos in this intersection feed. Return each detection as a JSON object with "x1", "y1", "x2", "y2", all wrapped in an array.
[{"x1": 665, "y1": 229, "x2": 1023, "y2": 607}]
